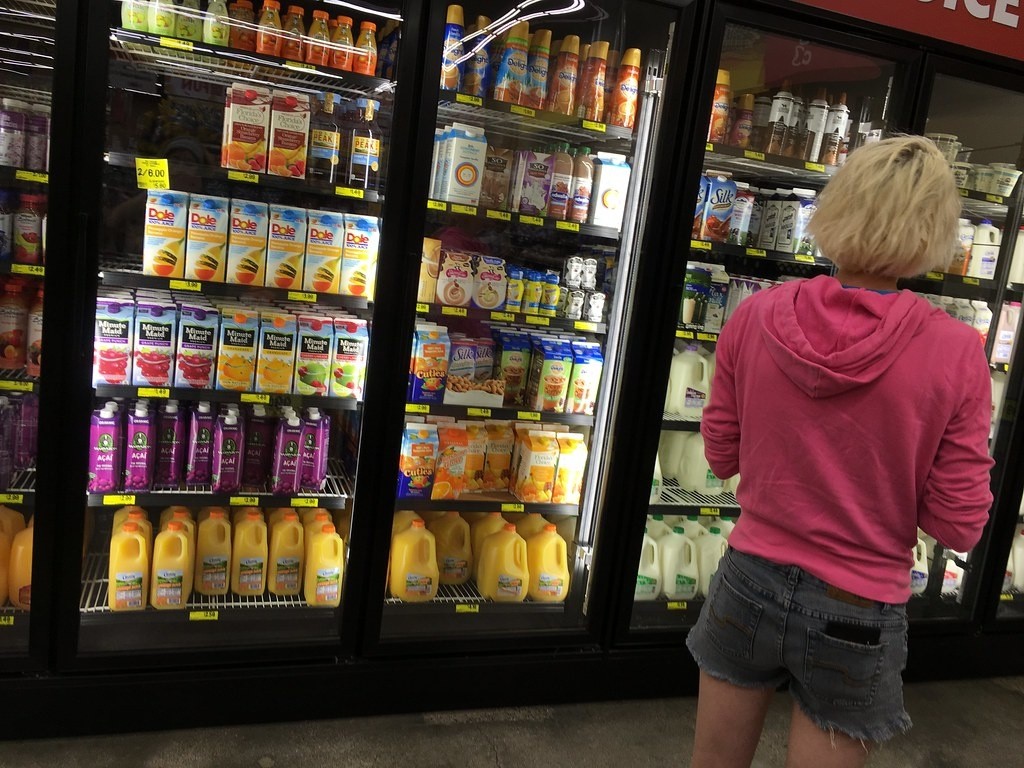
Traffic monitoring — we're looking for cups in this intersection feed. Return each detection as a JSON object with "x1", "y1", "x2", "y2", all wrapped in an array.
[{"x1": 927, "y1": 133, "x2": 1022, "y2": 197}]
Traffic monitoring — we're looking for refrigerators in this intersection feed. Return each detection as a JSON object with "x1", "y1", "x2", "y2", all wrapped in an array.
[{"x1": 0, "y1": 0, "x2": 1024, "y2": 736}]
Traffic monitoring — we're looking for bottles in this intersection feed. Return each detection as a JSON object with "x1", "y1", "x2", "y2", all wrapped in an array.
[
  {"x1": 306, "y1": 92, "x2": 382, "y2": 190},
  {"x1": 707, "y1": 69, "x2": 853, "y2": 165},
  {"x1": 505, "y1": 263, "x2": 560, "y2": 317},
  {"x1": 948, "y1": 219, "x2": 1024, "y2": 283},
  {"x1": 0, "y1": 99, "x2": 50, "y2": 608},
  {"x1": 647, "y1": 342, "x2": 742, "y2": 501},
  {"x1": 109, "y1": 510, "x2": 735, "y2": 612},
  {"x1": 529, "y1": 143, "x2": 594, "y2": 224},
  {"x1": 440, "y1": 4, "x2": 641, "y2": 128},
  {"x1": 991, "y1": 299, "x2": 1022, "y2": 363},
  {"x1": 121, "y1": 0, "x2": 398, "y2": 77},
  {"x1": 908, "y1": 522, "x2": 1024, "y2": 595}
]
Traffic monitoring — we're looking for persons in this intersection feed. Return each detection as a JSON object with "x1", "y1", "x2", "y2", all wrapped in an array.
[
  {"x1": 103, "y1": 133, "x2": 210, "y2": 257},
  {"x1": 686, "y1": 132, "x2": 994, "y2": 768}
]
